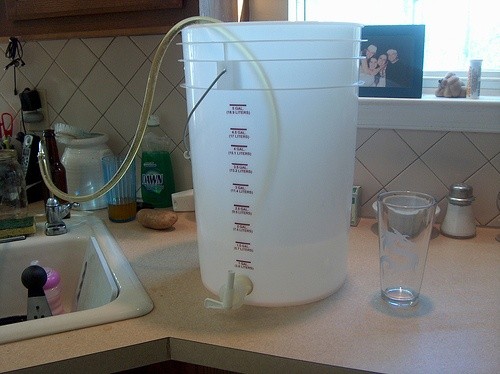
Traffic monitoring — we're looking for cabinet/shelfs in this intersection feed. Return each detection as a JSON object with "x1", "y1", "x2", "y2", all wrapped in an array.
[{"x1": 0, "y1": 0, "x2": 225, "y2": 44}]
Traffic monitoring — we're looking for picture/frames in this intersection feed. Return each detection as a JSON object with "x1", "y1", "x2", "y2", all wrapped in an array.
[{"x1": 357, "y1": 24, "x2": 426, "y2": 98}]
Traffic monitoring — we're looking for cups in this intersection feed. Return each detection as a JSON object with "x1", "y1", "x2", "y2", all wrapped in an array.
[
  {"x1": 377, "y1": 191, "x2": 438, "y2": 308},
  {"x1": 102, "y1": 155, "x2": 137, "y2": 223}
]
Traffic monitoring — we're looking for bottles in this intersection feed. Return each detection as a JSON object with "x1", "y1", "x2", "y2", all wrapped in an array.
[
  {"x1": 440, "y1": 184, "x2": 476, "y2": 239},
  {"x1": 60, "y1": 132, "x2": 116, "y2": 211},
  {"x1": 0, "y1": 149, "x2": 29, "y2": 220},
  {"x1": 42, "y1": 129, "x2": 67, "y2": 213},
  {"x1": 141, "y1": 115, "x2": 175, "y2": 207},
  {"x1": 41, "y1": 266, "x2": 63, "y2": 316}
]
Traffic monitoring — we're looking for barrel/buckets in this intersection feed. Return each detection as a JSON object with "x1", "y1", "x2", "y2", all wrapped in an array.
[{"x1": 174, "y1": 20, "x2": 369, "y2": 311}]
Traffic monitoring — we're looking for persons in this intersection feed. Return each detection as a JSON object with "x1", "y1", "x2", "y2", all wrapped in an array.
[{"x1": 359, "y1": 45, "x2": 407, "y2": 88}]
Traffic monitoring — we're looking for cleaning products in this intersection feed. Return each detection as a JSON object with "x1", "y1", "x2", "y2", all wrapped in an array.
[{"x1": 137, "y1": 114, "x2": 177, "y2": 207}]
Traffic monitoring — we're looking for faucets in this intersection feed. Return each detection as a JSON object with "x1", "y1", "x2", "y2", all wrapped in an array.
[{"x1": 43, "y1": 198, "x2": 80, "y2": 236}]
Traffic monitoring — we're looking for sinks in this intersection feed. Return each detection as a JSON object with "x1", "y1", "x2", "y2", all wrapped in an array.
[{"x1": 0, "y1": 207, "x2": 157, "y2": 346}]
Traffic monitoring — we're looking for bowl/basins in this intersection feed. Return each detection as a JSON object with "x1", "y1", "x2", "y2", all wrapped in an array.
[{"x1": 372, "y1": 196, "x2": 440, "y2": 236}]
